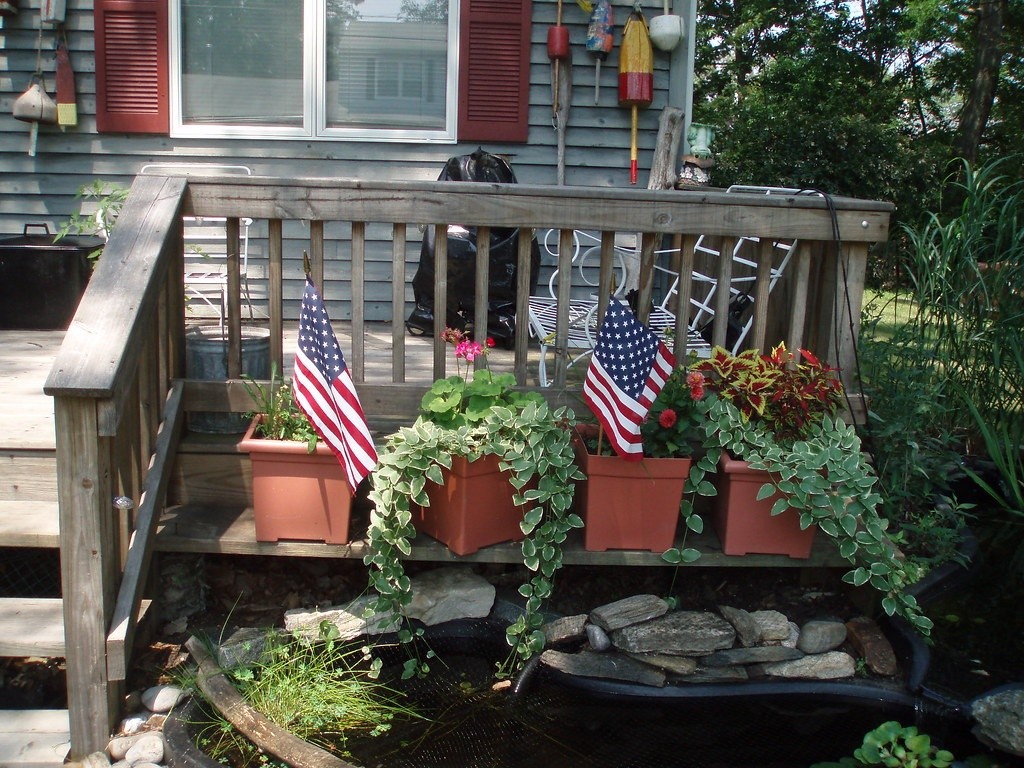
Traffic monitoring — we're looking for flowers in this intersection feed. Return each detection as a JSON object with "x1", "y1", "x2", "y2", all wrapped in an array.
[
  {"x1": 591, "y1": 324, "x2": 706, "y2": 485},
  {"x1": 362, "y1": 329, "x2": 589, "y2": 684}
]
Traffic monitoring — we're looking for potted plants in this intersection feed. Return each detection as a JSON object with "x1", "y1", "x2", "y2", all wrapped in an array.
[
  {"x1": 235, "y1": 361, "x2": 353, "y2": 546},
  {"x1": 662, "y1": 340, "x2": 936, "y2": 649}
]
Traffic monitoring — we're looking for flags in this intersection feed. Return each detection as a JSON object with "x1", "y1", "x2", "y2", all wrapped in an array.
[
  {"x1": 292, "y1": 273, "x2": 377, "y2": 498},
  {"x1": 581, "y1": 293, "x2": 677, "y2": 464}
]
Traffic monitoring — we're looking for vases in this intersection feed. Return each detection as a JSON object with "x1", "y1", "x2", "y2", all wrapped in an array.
[
  {"x1": 409, "y1": 451, "x2": 547, "y2": 557},
  {"x1": 563, "y1": 421, "x2": 694, "y2": 553}
]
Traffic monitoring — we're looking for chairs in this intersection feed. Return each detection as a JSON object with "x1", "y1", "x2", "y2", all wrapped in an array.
[{"x1": 513, "y1": 184, "x2": 825, "y2": 386}]
